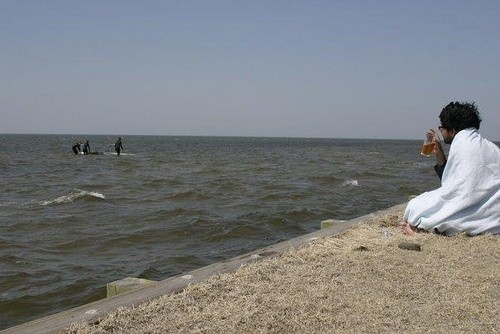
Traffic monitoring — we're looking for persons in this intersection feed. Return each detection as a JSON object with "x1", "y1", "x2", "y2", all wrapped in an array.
[
  {"x1": 114, "y1": 136, "x2": 125, "y2": 156},
  {"x1": 73, "y1": 141, "x2": 82, "y2": 156},
  {"x1": 398, "y1": 99, "x2": 500, "y2": 239},
  {"x1": 82, "y1": 140, "x2": 92, "y2": 156}
]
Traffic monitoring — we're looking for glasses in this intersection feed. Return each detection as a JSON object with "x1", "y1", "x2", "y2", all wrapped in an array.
[{"x1": 437, "y1": 123, "x2": 446, "y2": 129}]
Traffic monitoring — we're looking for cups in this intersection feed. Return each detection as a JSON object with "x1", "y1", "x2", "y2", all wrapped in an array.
[{"x1": 421, "y1": 134, "x2": 439, "y2": 158}]
